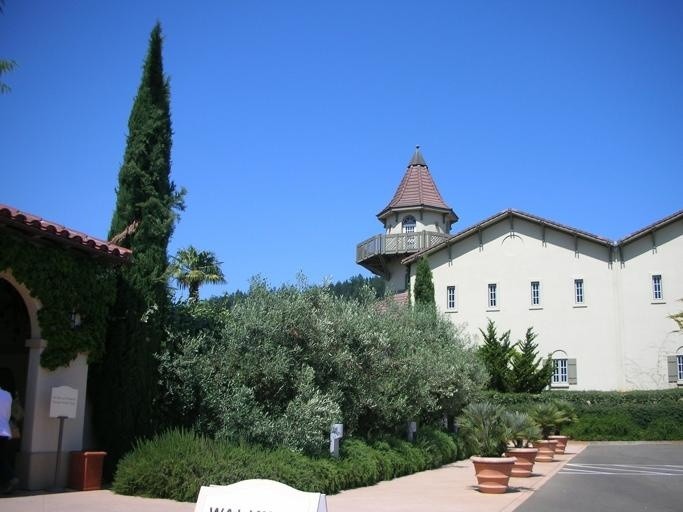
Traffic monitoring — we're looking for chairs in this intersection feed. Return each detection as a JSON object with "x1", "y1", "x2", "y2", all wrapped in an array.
[{"x1": 195, "y1": 479, "x2": 329, "y2": 512}]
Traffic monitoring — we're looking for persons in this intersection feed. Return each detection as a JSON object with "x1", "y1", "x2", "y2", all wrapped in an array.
[
  {"x1": 0, "y1": 373, "x2": 14, "y2": 498},
  {"x1": 7, "y1": 388, "x2": 25, "y2": 498}
]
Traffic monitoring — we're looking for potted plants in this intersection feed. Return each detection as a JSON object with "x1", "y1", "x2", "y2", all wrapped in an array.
[{"x1": 453, "y1": 399, "x2": 580, "y2": 495}]
